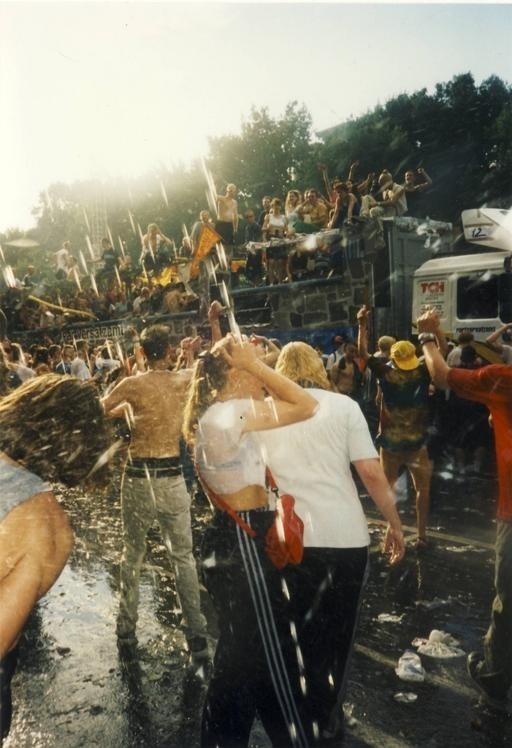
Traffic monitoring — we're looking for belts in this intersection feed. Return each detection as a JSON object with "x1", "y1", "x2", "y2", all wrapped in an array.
[{"x1": 122, "y1": 464, "x2": 184, "y2": 480}]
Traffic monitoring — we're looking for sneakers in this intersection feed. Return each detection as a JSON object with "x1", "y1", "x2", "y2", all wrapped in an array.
[
  {"x1": 464, "y1": 649, "x2": 510, "y2": 706},
  {"x1": 185, "y1": 634, "x2": 214, "y2": 665},
  {"x1": 117, "y1": 634, "x2": 140, "y2": 655}
]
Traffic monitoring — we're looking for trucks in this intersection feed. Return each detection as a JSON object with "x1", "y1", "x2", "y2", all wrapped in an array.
[{"x1": 24, "y1": 204, "x2": 512, "y2": 407}]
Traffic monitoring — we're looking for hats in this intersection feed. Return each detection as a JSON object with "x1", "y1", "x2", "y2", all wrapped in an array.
[{"x1": 390, "y1": 340, "x2": 423, "y2": 372}]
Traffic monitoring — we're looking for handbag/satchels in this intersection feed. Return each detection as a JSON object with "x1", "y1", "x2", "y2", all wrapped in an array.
[{"x1": 263, "y1": 492, "x2": 308, "y2": 569}]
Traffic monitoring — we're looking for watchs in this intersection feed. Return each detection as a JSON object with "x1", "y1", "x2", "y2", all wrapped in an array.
[{"x1": 418, "y1": 332, "x2": 435, "y2": 347}]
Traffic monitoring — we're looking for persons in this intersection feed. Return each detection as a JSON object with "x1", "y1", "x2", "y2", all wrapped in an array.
[
  {"x1": 251, "y1": 340, "x2": 407, "y2": 748},
  {"x1": 412, "y1": 306, "x2": 511, "y2": 711},
  {"x1": 97, "y1": 323, "x2": 211, "y2": 662},
  {"x1": 0, "y1": 372, "x2": 107, "y2": 662},
  {"x1": 182, "y1": 331, "x2": 322, "y2": 748},
  {"x1": 0, "y1": 159, "x2": 512, "y2": 548}
]
